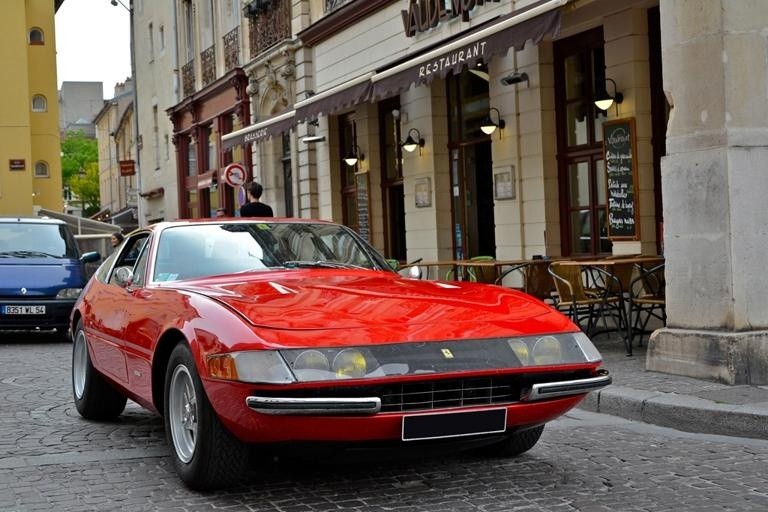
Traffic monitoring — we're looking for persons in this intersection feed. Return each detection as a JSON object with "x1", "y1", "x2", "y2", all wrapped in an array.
[
  {"x1": 216, "y1": 209, "x2": 228, "y2": 218},
  {"x1": 239, "y1": 182, "x2": 274, "y2": 216},
  {"x1": 110, "y1": 233, "x2": 123, "y2": 246}
]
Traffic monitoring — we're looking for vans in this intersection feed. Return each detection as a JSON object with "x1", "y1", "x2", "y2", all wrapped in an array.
[{"x1": 0, "y1": 217, "x2": 103, "y2": 339}]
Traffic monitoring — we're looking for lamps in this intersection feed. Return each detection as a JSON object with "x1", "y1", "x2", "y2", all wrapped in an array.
[
  {"x1": 594, "y1": 78, "x2": 623, "y2": 111},
  {"x1": 401, "y1": 128, "x2": 425, "y2": 153},
  {"x1": 478, "y1": 107, "x2": 505, "y2": 136},
  {"x1": 342, "y1": 144, "x2": 365, "y2": 167}
]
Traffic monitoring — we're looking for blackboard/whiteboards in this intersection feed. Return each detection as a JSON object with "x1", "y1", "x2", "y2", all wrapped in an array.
[{"x1": 354, "y1": 171, "x2": 373, "y2": 247}]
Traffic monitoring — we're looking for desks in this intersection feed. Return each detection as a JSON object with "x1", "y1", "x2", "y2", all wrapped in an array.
[{"x1": 400, "y1": 261, "x2": 467, "y2": 280}]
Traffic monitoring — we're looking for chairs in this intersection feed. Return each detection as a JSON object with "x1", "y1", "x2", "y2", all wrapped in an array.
[
  {"x1": 386, "y1": 259, "x2": 399, "y2": 271},
  {"x1": 408, "y1": 258, "x2": 422, "y2": 279},
  {"x1": 446, "y1": 256, "x2": 665, "y2": 358}
]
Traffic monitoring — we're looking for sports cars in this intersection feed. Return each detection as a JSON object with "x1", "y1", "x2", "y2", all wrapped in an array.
[{"x1": 67, "y1": 215, "x2": 614, "y2": 492}]
